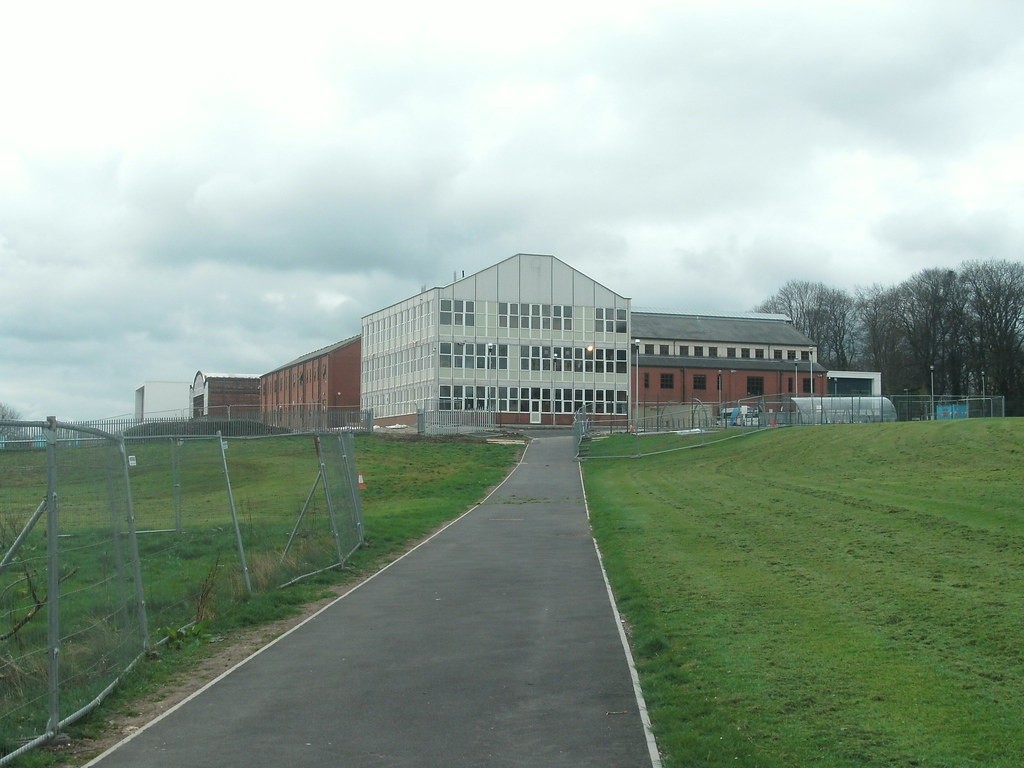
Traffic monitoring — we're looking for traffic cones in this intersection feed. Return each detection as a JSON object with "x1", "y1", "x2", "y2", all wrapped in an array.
[{"x1": 358, "y1": 471, "x2": 366, "y2": 489}]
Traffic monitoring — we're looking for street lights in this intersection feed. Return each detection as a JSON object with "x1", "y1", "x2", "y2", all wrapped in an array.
[
  {"x1": 982, "y1": 372, "x2": 985, "y2": 403},
  {"x1": 794, "y1": 359, "x2": 798, "y2": 398},
  {"x1": 487, "y1": 342, "x2": 493, "y2": 431},
  {"x1": 930, "y1": 365, "x2": 934, "y2": 421},
  {"x1": 634, "y1": 339, "x2": 641, "y2": 435},
  {"x1": 809, "y1": 346, "x2": 813, "y2": 395},
  {"x1": 553, "y1": 354, "x2": 557, "y2": 424}
]
